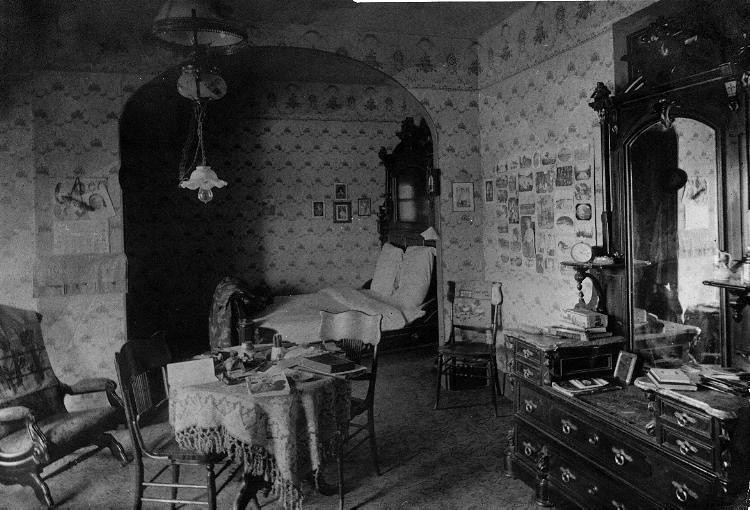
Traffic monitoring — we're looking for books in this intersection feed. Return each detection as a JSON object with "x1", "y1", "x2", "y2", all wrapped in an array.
[
  {"x1": 550, "y1": 376, "x2": 622, "y2": 398},
  {"x1": 549, "y1": 322, "x2": 614, "y2": 341},
  {"x1": 297, "y1": 354, "x2": 355, "y2": 373},
  {"x1": 244, "y1": 369, "x2": 292, "y2": 398},
  {"x1": 643, "y1": 361, "x2": 750, "y2": 397}
]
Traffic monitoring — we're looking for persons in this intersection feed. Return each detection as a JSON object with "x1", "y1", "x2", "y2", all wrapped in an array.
[
  {"x1": 315, "y1": 205, "x2": 323, "y2": 216},
  {"x1": 337, "y1": 188, "x2": 346, "y2": 199},
  {"x1": 339, "y1": 207, "x2": 346, "y2": 220},
  {"x1": 456, "y1": 188, "x2": 468, "y2": 207},
  {"x1": 523, "y1": 218, "x2": 536, "y2": 257}
]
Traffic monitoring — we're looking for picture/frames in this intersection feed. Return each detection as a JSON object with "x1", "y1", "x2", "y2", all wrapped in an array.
[
  {"x1": 357, "y1": 197, "x2": 372, "y2": 217},
  {"x1": 311, "y1": 200, "x2": 326, "y2": 220},
  {"x1": 333, "y1": 182, "x2": 349, "y2": 201},
  {"x1": 332, "y1": 201, "x2": 353, "y2": 224}
]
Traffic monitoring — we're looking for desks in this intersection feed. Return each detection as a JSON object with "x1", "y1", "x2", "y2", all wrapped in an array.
[
  {"x1": 683, "y1": 301, "x2": 722, "y2": 356},
  {"x1": 165, "y1": 342, "x2": 355, "y2": 510}
]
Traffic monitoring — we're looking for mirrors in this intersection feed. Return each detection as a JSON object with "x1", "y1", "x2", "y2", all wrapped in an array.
[{"x1": 621, "y1": 109, "x2": 729, "y2": 392}]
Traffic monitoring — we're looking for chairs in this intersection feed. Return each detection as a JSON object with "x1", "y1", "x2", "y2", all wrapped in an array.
[
  {"x1": 0, "y1": 304, "x2": 131, "y2": 510},
  {"x1": 114, "y1": 331, "x2": 260, "y2": 510},
  {"x1": 319, "y1": 304, "x2": 387, "y2": 508},
  {"x1": 434, "y1": 278, "x2": 503, "y2": 415}
]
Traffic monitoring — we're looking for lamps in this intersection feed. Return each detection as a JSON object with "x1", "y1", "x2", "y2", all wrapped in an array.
[{"x1": 148, "y1": 2, "x2": 247, "y2": 209}]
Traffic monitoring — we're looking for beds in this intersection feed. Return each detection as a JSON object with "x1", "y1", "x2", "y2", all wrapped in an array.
[{"x1": 211, "y1": 240, "x2": 434, "y2": 355}]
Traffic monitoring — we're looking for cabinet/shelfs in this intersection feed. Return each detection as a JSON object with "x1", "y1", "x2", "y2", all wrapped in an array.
[
  {"x1": 632, "y1": 310, "x2": 701, "y2": 368},
  {"x1": 507, "y1": 256, "x2": 749, "y2": 509},
  {"x1": 374, "y1": 115, "x2": 434, "y2": 240}
]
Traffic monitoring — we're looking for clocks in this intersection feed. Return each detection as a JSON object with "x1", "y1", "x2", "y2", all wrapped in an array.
[{"x1": 571, "y1": 240, "x2": 595, "y2": 262}]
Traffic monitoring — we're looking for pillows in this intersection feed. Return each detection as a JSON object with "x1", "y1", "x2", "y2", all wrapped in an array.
[
  {"x1": 368, "y1": 241, "x2": 402, "y2": 298},
  {"x1": 388, "y1": 242, "x2": 434, "y2": 309}
]
